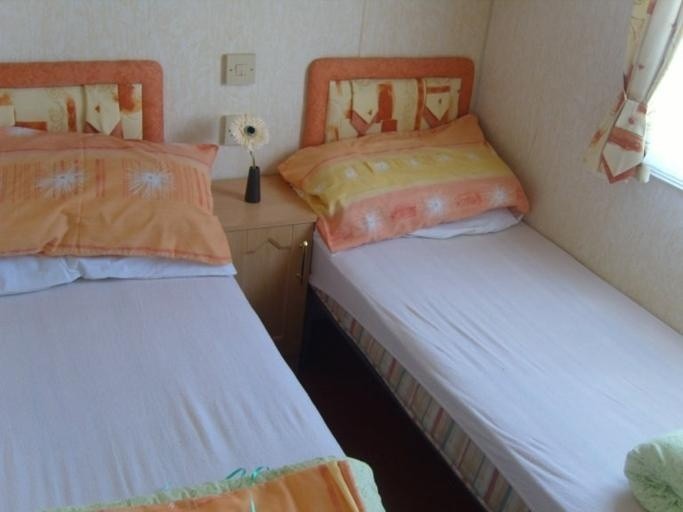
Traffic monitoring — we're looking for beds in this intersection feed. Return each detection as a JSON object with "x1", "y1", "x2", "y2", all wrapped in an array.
[
  {"x1": 1, "y1": 58, "x2": 385, "y2": 512},
  {"x1": 300, "y1": 58, "x2": 683, "y2": 512}
]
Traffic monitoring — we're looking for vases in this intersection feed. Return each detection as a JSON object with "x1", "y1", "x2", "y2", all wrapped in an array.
[{"x1": 244, "y1": 165, "x2": 261, "y2": 202}]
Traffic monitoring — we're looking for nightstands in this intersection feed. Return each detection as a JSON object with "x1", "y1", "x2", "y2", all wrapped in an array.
[{"x1": 213, "y1": 173, "x2": 318, "y2": 362}]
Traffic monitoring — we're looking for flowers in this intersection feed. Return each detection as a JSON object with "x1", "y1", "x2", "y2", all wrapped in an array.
[{"x1": 229, "y1": 116, "x2": 269, "y2": 169}]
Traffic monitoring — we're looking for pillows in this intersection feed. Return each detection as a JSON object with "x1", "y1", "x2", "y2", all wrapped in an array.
[
  {"x1": 414, "y1": 208, "x2": 523, "y2": 239},
  {"x1": 277, "y1": 114, "x2": 529, "y2": 255},
  {"x1": 0, "y1": 257, "x2": 237, "y2": 295},
  {"x1": 0, "y1": 133, "x2": 233, "y2": 266}
]
